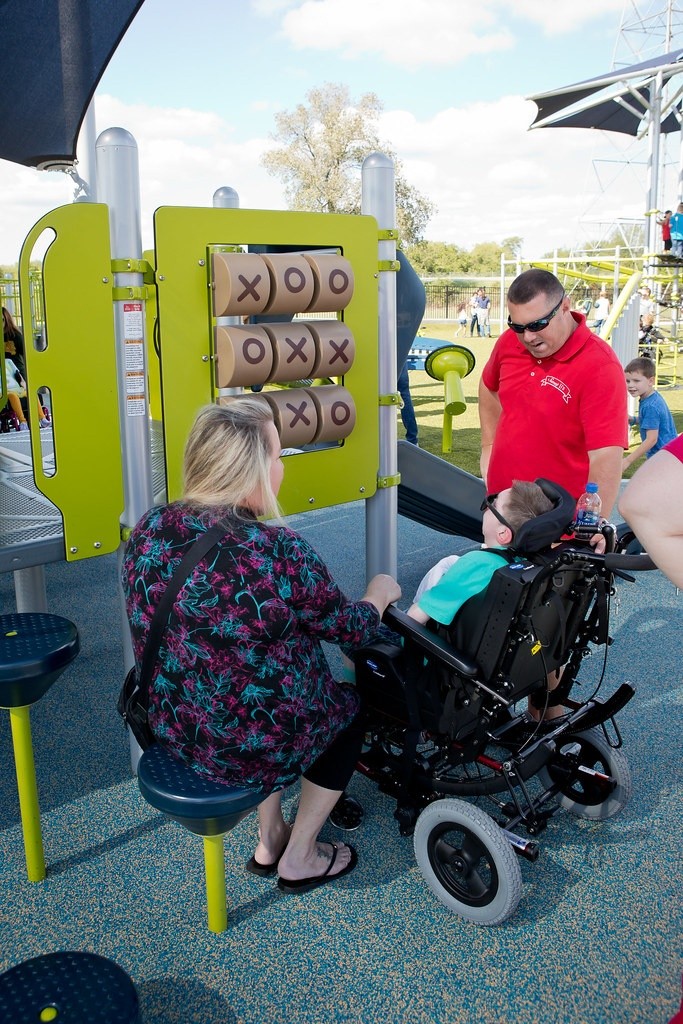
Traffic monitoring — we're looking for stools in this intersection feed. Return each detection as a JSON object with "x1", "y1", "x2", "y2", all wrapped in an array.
[
  {"x1": 0, "y1": 612, "x2": 82, "y2": 882},
  {"x1": 0, "y1": 951, "x2": 141, "y2": 1024},
  {"x1": 137, "y1": 752, "x2": 263, "y2": 934}
]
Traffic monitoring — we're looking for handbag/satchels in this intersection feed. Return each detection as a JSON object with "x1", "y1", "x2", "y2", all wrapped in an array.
[{"x1": 116, "y1": 666, "x2": 155, "y2": 751}]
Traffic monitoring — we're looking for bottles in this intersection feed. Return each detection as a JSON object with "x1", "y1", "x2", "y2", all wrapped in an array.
[{"x1": 576, "y1": 483, "x2": 602, "y2": 537}]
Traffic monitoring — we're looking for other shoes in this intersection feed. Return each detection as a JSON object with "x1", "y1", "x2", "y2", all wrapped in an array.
[
  {"x1": 40, "y1": 418, "x2": 51, "y2": 428},
  {"x1": 20, "y1": 422, "x2": 30, "y2": 431}
]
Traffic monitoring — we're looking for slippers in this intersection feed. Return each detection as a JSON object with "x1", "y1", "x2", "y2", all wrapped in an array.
[
  {"x1": 278, "y1": 842, "x2": 358, "y2": 894},
  {"x1": 245, "y1": 840, "x2": 287, "y2": 877}
]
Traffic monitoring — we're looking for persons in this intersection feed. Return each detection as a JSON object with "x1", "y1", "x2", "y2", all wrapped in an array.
[
  {"x1": 617, "y1": 432, "x2": 683, "y2": 591},
  {"x1": 0, "y1": 307, "x2": 52, "y2": 430},
  {"x1": 594, "y1": 292, "x2": 611, "y2": 340},
  {"x1": 122, "y1": 399, "x2": 401, "y2": 894},
  {"x1": 656, "y1": 201, "x2": 683, "y2": 257},
  {"x1": 622, "y1": 357, "x2": 679, "y2": 473},
  {"x1": 406, "y1": 478, "x2": 554, "y2": 625},
  {"x1": 478, "y1": 269, "x2": 629, "y2": 555},
  {"x1": 454, "y1": 286, "x2": 491, "y2": 337},
  {"x1": 638, "y1": 287, "x2": 669, "y2": 363}
]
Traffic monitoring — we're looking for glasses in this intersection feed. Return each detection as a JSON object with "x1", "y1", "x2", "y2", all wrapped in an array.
[
  {"x1": 481, "y1": 494, "x2": 515, "y2": 544},
  {"x1": 506, "y1": 291, "x2": 565, "y2": 333}
]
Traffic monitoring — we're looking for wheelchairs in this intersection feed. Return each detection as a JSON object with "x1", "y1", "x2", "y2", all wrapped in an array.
[
  {"x1": 342, "y1": 477, "x2": 658, "y2": 928},
  {"x1": 0, "y1": 387, "x2": 50, "y2": 433}
]
